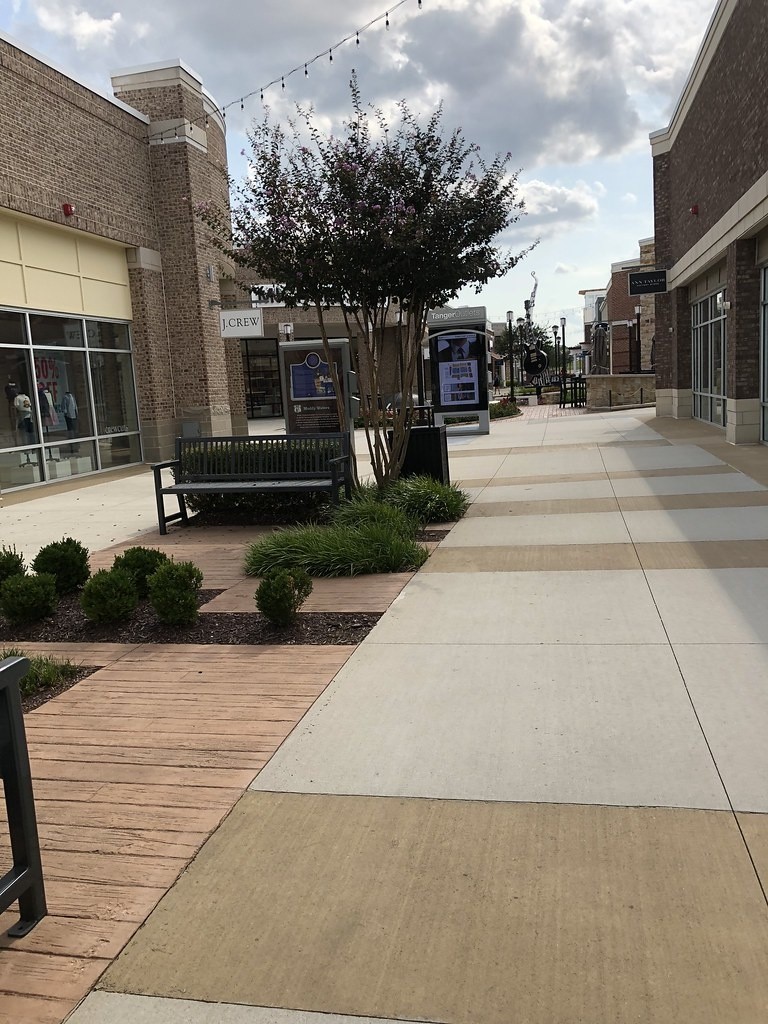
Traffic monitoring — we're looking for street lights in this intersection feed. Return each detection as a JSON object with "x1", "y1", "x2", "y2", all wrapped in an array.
[
  {"x1": 552, "y1": 325, "x2": 559, "y2": 382},
  {"x1": 560, "y1": 318, "x2": 566, "y2": 389},
  {"x1": 284, "y1": 324, "x2": 291, "y2": 342},
  {"x1": 517, "y1": 317, "x2": 525, "y2": 386},
  {"x1": 556, "y1": 336, "x2": 561, "y2": 370},
  {"x1": 507, "y1": 311, "x2": 514, "y2": 398},
  {"x1": 635, "y1": 304, "x2": 642, "y2": 373},
  {"x1": 395, "y1": 311, "x2": 404, "y2": 392}
]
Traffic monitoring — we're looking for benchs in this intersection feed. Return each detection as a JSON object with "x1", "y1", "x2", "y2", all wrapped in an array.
[{"x1": 150, "y1": 430, "x2": 351, "y2": 536}]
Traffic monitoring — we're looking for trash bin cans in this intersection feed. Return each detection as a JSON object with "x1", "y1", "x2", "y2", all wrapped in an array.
[{"x1": 386, "y1": 405, "x2": 451, "y2": 487}]
